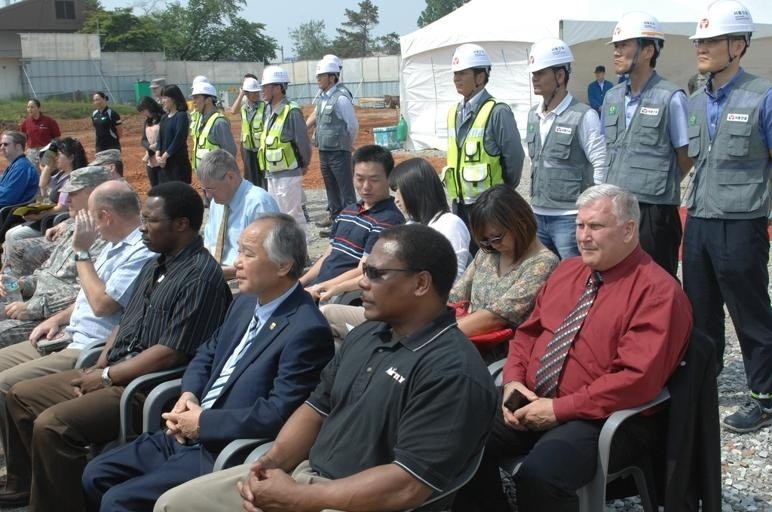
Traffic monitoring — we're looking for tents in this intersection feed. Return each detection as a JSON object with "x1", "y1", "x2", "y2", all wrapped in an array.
[{"x1": 398, "y1": 0, "x2": 770, "y2": 156}]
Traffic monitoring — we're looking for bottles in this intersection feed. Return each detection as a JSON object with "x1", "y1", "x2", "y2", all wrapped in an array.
[
  {"x1": 3, "y1": 267, "x2": 24, "y2": 305},
  {"x1": 317, "y1": 291, "x2": 332, "y2": 310}
]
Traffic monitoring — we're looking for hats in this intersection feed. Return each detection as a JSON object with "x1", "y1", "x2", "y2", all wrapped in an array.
[
  {"x1": 39, "y1": 142, "x2": 55, "y2": 158},
  {"x1": 149, "y1": 78, "x2": 165, "y2": 88},
  {"x1": 87, "y1": 149, "x2": 122, "y2": 166},
  {"x1": 57, "y1": 166, "x2": 113, "y2": 192},
  {"x1": 594, "y1": 66, "x2": 605, "y2": 73}
]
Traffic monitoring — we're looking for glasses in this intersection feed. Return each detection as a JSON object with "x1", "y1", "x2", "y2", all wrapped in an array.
[
  {"x1": 362, "y1": 263, "x2": 412, "y2": 280},
  {"x1": 479, "y1": 231, "x2": 505, "y2": 247},
  {"x1": 200, "y1": 186, "x2": 216, "y2": 193},
  {"x1": 0, "y1": 142, "x2": 9, "y2": 147},
  {"x1": 693, "y1": 38, "x2": 717, "y2": 47},
  {"x1": 139, "y1": 216, "x2": 159, "y2": 225}
]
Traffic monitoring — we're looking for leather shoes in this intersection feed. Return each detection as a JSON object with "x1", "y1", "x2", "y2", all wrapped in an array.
[{"x1": 1, "y1": 474, "x2": 31, "y2": 507}]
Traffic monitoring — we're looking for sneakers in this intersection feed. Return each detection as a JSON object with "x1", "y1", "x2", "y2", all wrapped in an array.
[{"x1": 722, "y1": 396, "x2": 771, "y2": 432}]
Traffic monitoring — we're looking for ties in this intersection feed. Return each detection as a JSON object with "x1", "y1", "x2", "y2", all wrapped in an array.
[
  {"x1": 214, "y1": 204, "x2": 230, "y2": 265},
  {"x1": 533, "y1": 270, "x2": 603, "y2": 398},
  {"x1": 200, "y1": 310, "x2": 259, "y2": 411}
]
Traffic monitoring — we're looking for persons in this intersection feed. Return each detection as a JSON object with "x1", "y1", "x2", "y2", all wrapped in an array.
[
  {"x1": 91, "y1": 92, "x2": 121, "y2": 154},
  {"x1": 443, "y1": 1, "x2": 771, "y2": 433},
  {"x1": 136, "y1": 54, "x2": 359, "y2": 243},
  {"x1": 0, "y1": 131, "x2": 694, "y2": 512},
  {"x1": 21, "y1": 99, "x2": 61, "y2": 165}
]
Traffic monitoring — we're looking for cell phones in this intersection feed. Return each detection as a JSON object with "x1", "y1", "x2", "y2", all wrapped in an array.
[{"x1": 504, "y1": 388, "x2": 531, "y2": 413}]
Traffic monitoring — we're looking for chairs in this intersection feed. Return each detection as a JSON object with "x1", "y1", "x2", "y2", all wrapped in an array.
[
  {"x1": 299, "y1": 266, "x2": 313, "y2": 278},
  {"x1": 0, "y1": 195, "x2": 39, "y2": 224},
  {"x1": 38, "y1": 212, "x2": 61, "y2": 236},
  {"x1": 76, "y1": 346, "x2": 106, "y2": 369},
  {"x1": 74, "y1": 336, "x2": 110, "y2": 360},
  {"x1": 330, "y1": 289, "x2": 360, "y2": 305},
  {"x1": 244, "y1": 440, "x2": 486, "y2": 512},
  {"x1": 485, "y1": 328, "x2": 714, "y2": 512},
  {"x1": 142, "y1": 376, "x2": 187, "y2": 436},
  {"x1": 116, "y1": 366, "x2": 188, "y2": 445},
  {"x1": 53, "y1": 212, "x2": 69, "y2": 226},
  {"x1": 212, "y1": 433, "x2": 271, "y2": 472}
]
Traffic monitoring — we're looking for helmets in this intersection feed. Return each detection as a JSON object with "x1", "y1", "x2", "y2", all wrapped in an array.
[
  {"x1": 316, "y1": 54, "x2": 343, "y2": 74},
  {"x1": 688, "y1": 0, "x2": 755, "y2": 40},
  {"x1": 261, "y1": 65, "x2": 291, "y2": 87},
  {"x1": 523, "y1": 37, "x2": 574, "y2": 72},
  {"x1": 242, "y1": 77, "x2": 262, "y2": 93},
  {"x1": 605, "y1": 11, "x2": 664, "y2": 46},
  {"x1": 188, "y1": 76, "x2": 217, "y2": 100},
  {"x1": 451, "y1": 43, "x2": 492, "y2": 73}
]
